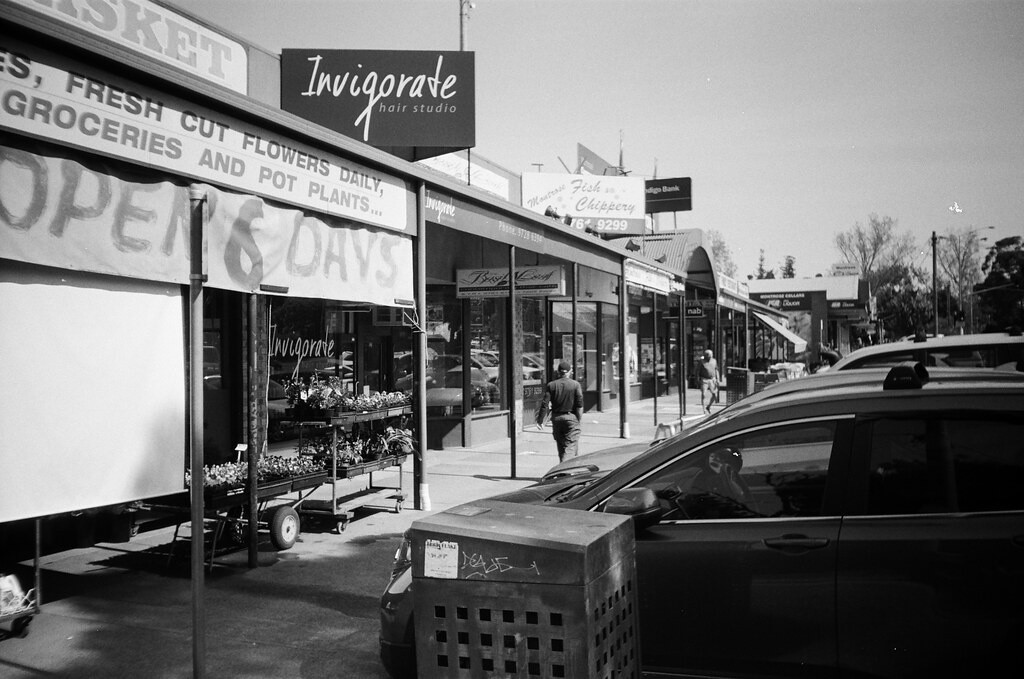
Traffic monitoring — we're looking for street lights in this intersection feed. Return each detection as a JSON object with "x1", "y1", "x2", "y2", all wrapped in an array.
[{"x1": 931, "y1": 225, "x2": 996, "y2": 337}]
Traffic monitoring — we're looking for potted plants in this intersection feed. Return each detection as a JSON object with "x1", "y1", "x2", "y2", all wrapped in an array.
[
  {"x1": 282, "y1": 371, "x2": 347, "y2": 425},
  {"x1": 373, "y1": 433, "x2": 389, "y2": 460}
]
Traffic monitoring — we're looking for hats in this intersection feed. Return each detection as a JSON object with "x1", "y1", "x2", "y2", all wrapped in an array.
[{"x1": 558, "y1": 362, "x2": 571, "y2": 373}]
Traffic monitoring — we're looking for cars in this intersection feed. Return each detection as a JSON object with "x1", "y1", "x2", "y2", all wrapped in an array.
[
  {"x1": 376, "y1": 379, "x2": 1024, "y2": 679},
  {"x1": 332, "y1": 345, "x2": 615, "y2": 420},
  {"x1": 539, "y1": 366, "x2": 1024, "y2": 482},
  {"x1": 206, "y1": 374, "x2": 295, "y2": 418},
  {"x1": 825, "y1": 335, "x2": 1024, "y2": 373},
  {"x1": 898, "y1": 334, "x2": 944, "y2": 342}
]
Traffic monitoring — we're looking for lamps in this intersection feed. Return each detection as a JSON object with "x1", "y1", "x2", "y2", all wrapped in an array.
[
  {"x1": 655, "y1": 253, "x2": 666, "y2": 264},
  {"x1": 625, "y1": 238, "x2": 640, "y2": 252},
  {"x1": 585, "y1": 224, "x2": 599, "y2": 237},
  {"x1": 544, "y1": 204, "x2": 560, "y2": 220}
]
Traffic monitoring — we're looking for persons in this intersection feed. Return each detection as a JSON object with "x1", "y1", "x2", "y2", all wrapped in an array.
[
  {"x1": 696, "y1": 350, "x2": 719, "y2": 413},
  {"x1": 913, "y1": 325, "x2": 928, "y2": 365},
  {"x1": 535, "y1": 362, "x2": 582, "y2": 465}
]
